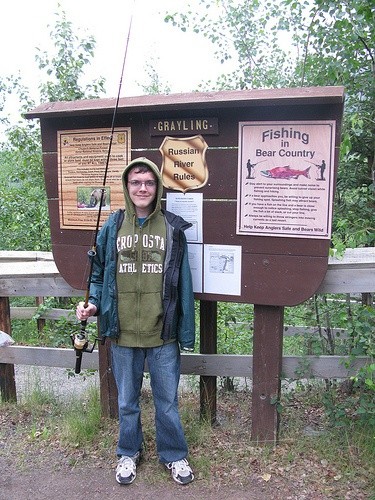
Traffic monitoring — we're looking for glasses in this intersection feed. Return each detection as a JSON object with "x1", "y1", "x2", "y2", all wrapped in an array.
[{"x1": 128, "y1": 180, "x2": 157, "y2": 188}]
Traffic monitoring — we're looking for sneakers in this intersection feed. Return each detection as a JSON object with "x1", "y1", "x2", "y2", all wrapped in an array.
[
  {"x1": 164, "y1": 457, "x2": 195, "y2": 484},
  {"x1": 116, "y1": 451, "x2": 140, "y2": 484}
]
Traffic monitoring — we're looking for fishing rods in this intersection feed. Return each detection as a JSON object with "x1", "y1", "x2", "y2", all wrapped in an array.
[{"x1": 69, "y1": 15, "x2": 132, "y2": 374}]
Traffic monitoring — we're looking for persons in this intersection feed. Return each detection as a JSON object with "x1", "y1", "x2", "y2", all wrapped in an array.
[{"x1": 77, "y1": 156, "x2": 196, "y2": 485}]
